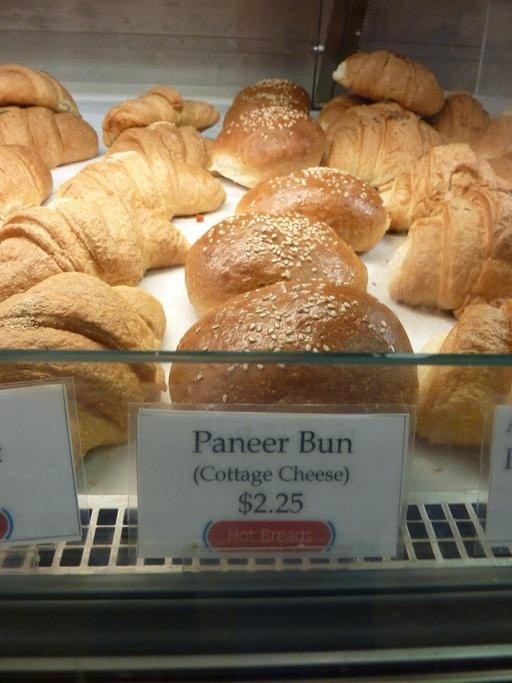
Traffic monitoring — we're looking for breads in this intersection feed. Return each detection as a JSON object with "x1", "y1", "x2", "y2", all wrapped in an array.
[{"x1": 0, "y1": 51, "x2": 512, "y2": 469}]
